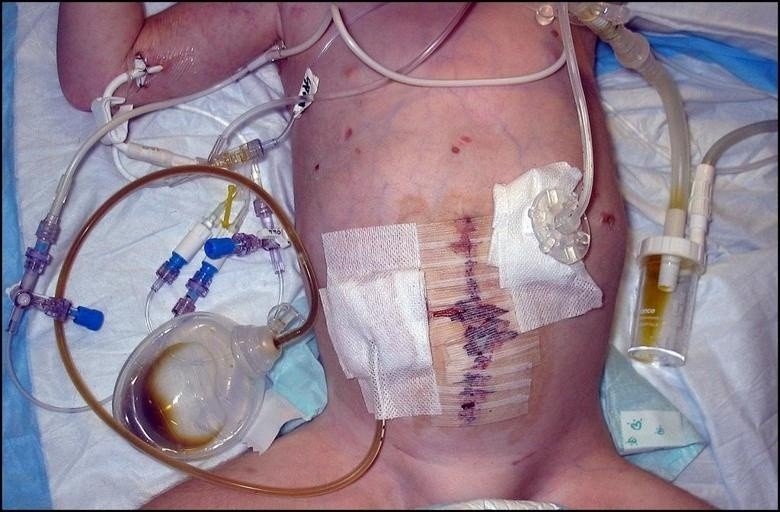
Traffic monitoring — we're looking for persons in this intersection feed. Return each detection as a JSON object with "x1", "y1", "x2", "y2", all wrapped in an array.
[{"x1": 56, "y1": 0, "x2": 717, "y2": 509}]
